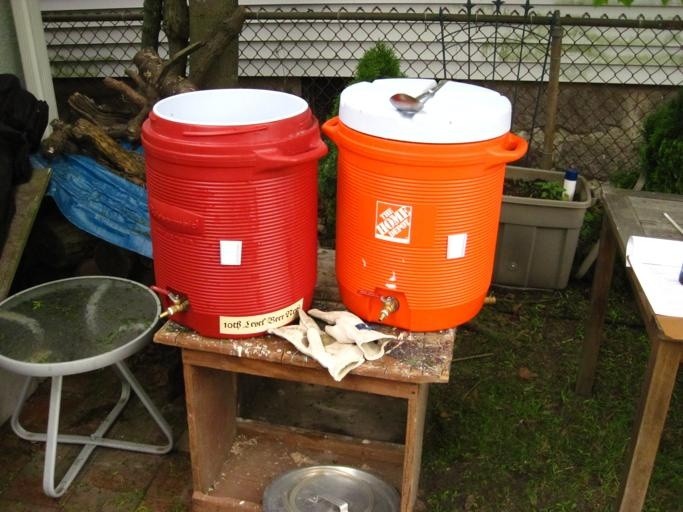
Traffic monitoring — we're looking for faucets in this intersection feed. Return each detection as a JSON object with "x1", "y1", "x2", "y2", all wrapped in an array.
[
  {"x1": 358, "y1": 289, "x2": 400, "y2": 322},
  {"x1": 149, "y1": 285, "x2": 190, "y2": 319}
]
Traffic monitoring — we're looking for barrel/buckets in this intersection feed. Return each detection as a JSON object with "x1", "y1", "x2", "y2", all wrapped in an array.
[
  {"x1": 320, "y1": 78, "x2": 529, "y2": 333},
  {"x1": 141, "y1": 88, "x2": 329, "y2": 339}
]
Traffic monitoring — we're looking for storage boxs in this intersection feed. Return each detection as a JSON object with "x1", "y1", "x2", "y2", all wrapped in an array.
[{"x1": 490, "y1": 165, "x2": 591, "y2": 290}]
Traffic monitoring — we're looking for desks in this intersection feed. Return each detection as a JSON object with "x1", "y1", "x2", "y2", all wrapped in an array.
[{"x1": 578, "y1": 185, "x2": 683, "y2": 511}]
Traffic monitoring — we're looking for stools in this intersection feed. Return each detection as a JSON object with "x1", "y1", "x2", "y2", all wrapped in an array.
[
  {"x1": 1, "y1": 275, "x2": 175, "y2": 497},
  {"x1": 154, "y1": 246, "x2": 459, "y2": 512}
]
